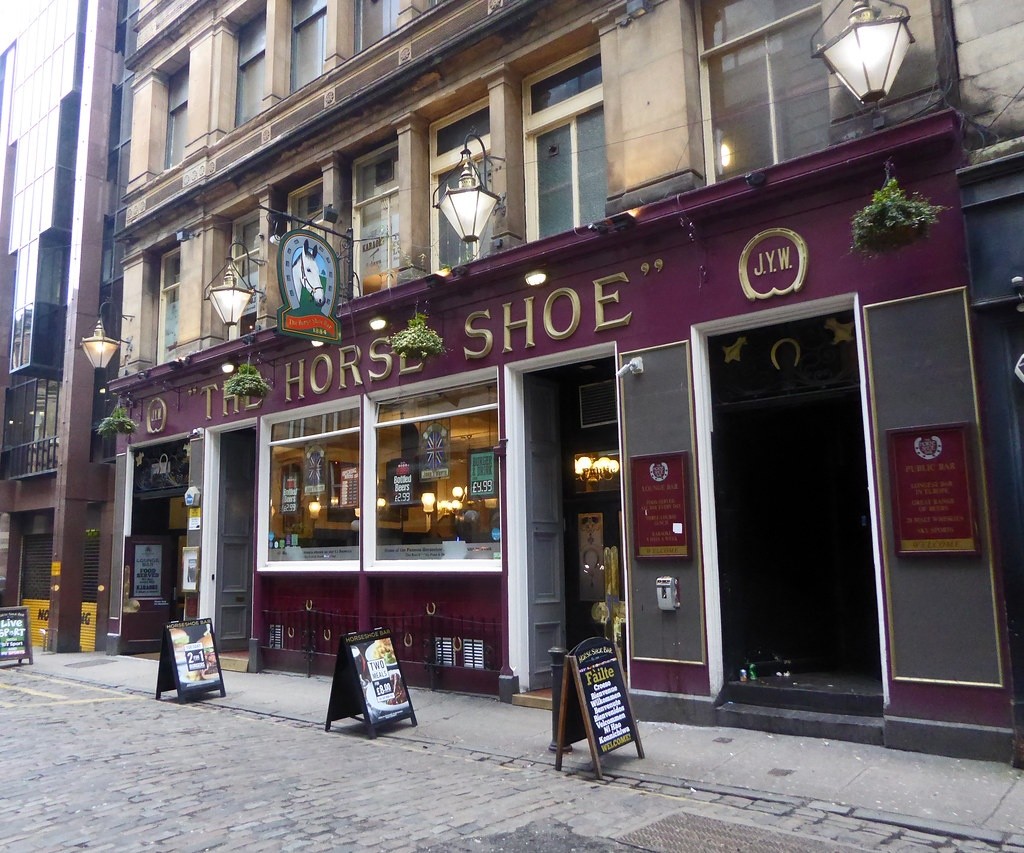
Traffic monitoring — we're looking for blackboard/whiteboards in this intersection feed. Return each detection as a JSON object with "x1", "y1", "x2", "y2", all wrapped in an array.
[
  {"x1": 570, "y1": 636, "x2": 638, "y2": 758},
  {"x1": 0, "y1": 606, "x2": 33, "y2": 661}
]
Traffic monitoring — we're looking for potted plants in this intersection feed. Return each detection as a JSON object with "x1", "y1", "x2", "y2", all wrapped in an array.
[
  {"x1": 94, "y1": 407, "x2": 138, "y2": 438},
  {"x1": 387, "y1": 312, "x2": 454, "y2": 367},
  {"x1": 847, "y1": 178, "x2": 955, "y2": 258},
  {"x1": 224, "y1": 363, "x2": 274, "y2": 399}
]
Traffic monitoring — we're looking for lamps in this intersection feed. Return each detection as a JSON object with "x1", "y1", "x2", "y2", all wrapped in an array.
[
  {"x1": 309, "y1": 501, "x2": 321, "y2": 521},
  {"x1": 524, "y1": 260, "x2": 547, "y2": 286},
  {"x1": 368, "y1": 316, "x2": 388, "y2": 331},
  {"x1": 355, "y1": 507, "x2": 360, "y2": 518},
  {"x1": 627, "y1": 0, "x2": 653, "y2": 19},
  {"x1": 421, "y1": 493, "x2": 435, "y2": 517},
  {"x1": 574, "y1": 452, "x2": 620, "y2": 484},
  {"x1": 204, "y1": 241, "x2": 266, "y2": 325},
  {"x1": 451, "y1": 433, "x2": 484, "y2": 512},
  {"x1": 311, "y1": 204, "x2": 337, "y2": 226},
  {"x1": 222, "y1": 362, "x2": 234, "y2": 373},
  {"x1": 376, "y1": 498, "x2": 386, "y2": 511},
  {"x1": 79, "y1": 296, "x2": 135, "y2": 371},
  {"x1": 177, "y1": 232, "x2": 197, "y2": 242},
  {"x1": 810, "y1": 0, "x2": 915, "y2": 131},
  {"x1": 433, "y1": 125, "x2": 507, "y2": 243}
]
